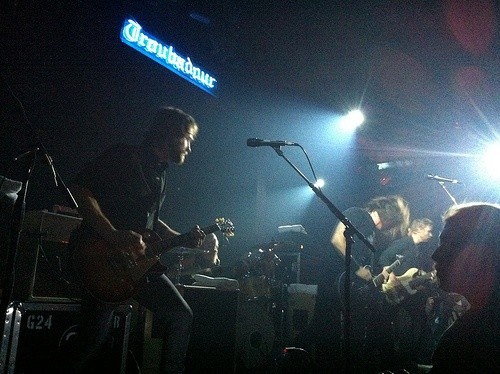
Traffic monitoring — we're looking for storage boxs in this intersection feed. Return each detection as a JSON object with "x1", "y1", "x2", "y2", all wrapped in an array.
[{"x1": 0, "y1": 301, "x2": 133, "y2": 374}]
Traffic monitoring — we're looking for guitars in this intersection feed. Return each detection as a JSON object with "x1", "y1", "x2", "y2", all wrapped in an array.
[
  {"x1": 72, "y1": 216, "x2": 236, "y2": 305},
  {"x1": 382, "y1": 266, "x2": 440, "y2": 305},
  {"x1": 336, "y1": 252, "x2": 404, "y2": 321}
]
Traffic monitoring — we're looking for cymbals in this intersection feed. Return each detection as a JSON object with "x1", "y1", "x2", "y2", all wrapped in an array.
[
  {"x1": 165, "y1": 246, "x2": 208, "y2": 255},
  {"x1": 254, "y1": 239, "x2": 295, "y2": 252}
]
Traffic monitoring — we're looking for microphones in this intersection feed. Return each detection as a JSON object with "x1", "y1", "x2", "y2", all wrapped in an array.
[
  {"x1": 426, "y1": 175, "x2": 464, "y2": 185},
  {"x1": 247, "y1": 137, "x2": 299, "y2": 146}
]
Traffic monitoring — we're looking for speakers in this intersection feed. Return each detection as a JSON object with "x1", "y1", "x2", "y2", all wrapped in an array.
[
  {"x1": 14, "y1": 209, "x2": 82, "y2": 304},
  {"x1": 175, "y1": 281, "x2": 238, "y2": 374},
  {"x1": 0, "y1": 301, "x2": 132, "y2": 374}
]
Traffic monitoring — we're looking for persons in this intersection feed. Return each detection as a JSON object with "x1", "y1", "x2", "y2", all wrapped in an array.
[
  {"x1": 192, "y1": 231, "x2": 220, "y2": 270},
  {"x1": 58, "y1": 108, "x2": 205, "y2": 374},
  {"x1": 328, "y1": 195, "x2": 500, "y2": 374}
]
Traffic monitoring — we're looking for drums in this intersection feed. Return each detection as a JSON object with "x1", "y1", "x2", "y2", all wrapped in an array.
[
  {"x1": 280, "y1": 282, "x2": 319, "y2": 368},
  {"x1": 234, "y1": 303, "x2": 276, "y2": 368},
  {"x1": 237, "y1": 271, "x2": 277, "y2": 309}
]
